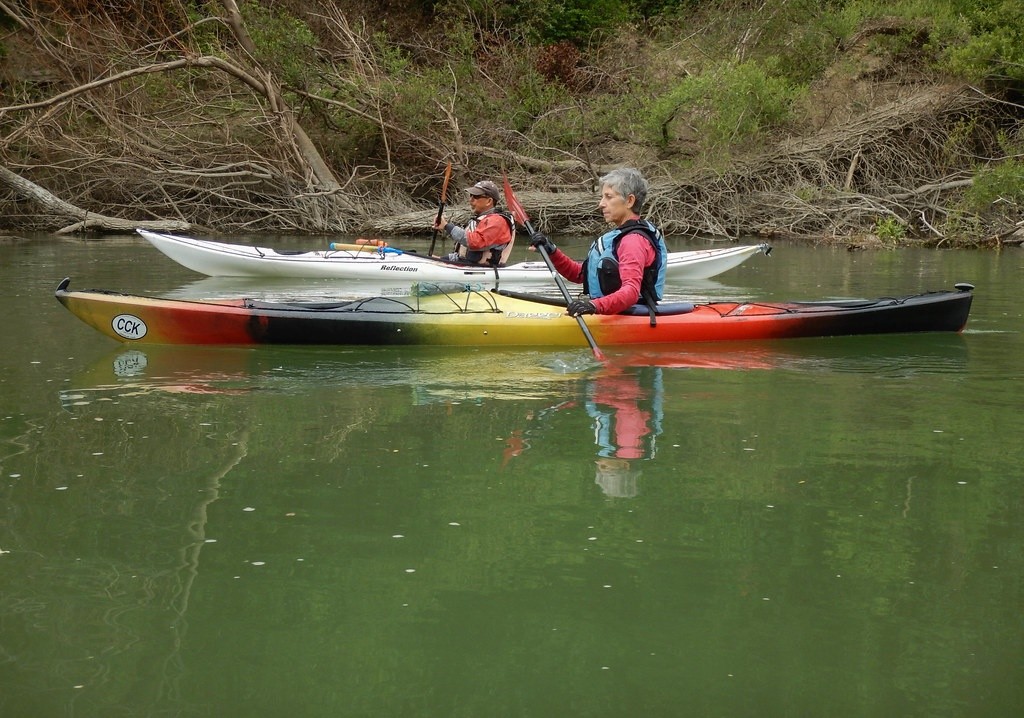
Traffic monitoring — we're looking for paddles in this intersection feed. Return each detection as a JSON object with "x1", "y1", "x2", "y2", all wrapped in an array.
[
  {"x1": 427, "y1": 161, "x2": 451, "y2": 255},
  {"x1": 503, "y1": 172, "x2": 607, "y2": 362}
]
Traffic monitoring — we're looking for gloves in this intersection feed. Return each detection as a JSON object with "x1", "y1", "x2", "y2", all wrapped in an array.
[
  {"x1": 567, "y1": 301, "x2": 596, "y2": 317},
  {"x1": 528, "y1": 232, "x2": 557, "y2": 255}
]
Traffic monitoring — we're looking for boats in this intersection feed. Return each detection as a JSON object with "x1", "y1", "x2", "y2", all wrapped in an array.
[
  {"x1": 54, "y1": 282, "x2": 976, "y2": 346},
  {"x1": 135, "y1": 227, "x2": 773, "y2": 283}
]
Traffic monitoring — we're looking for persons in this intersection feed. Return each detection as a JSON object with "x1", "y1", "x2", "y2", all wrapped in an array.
[
  {"x1": 529, "y1": 168, "x2": 667, "y2": 316},
  {"x1": 427, "y1": 180, "x2": 515, "y2": 268}
]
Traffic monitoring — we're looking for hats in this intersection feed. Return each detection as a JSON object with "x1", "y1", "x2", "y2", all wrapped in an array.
[{"x1": 464, "y1": 181, "x2": 499, "y2": 201}]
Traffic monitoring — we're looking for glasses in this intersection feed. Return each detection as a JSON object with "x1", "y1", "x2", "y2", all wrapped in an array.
[{"x1": 470, "y1": 193, "x2": 490, "y2": 199}]
